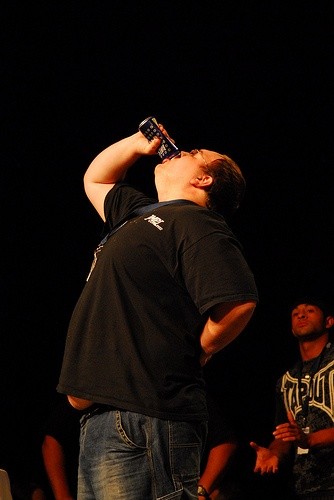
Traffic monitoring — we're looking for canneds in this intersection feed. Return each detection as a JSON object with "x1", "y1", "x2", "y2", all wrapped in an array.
[{"x1": 139, "y1": 117, "x2": 181, "y2": 160}]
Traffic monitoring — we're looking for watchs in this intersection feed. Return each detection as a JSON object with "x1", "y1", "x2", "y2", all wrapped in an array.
[{"x1": 196, "y1": 485, "x2": 209, "y2": 499}]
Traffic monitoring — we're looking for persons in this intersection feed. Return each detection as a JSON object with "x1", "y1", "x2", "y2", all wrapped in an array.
[
  {"x1": 56, "y1": 124, "x2": 260, "y2": 500},
  {"x1": 249, "y1": 296, "x2": 334, "y2": 500},
  {"x1": 43, "y1": 405, "x2": 82, "y2": 500}
]
